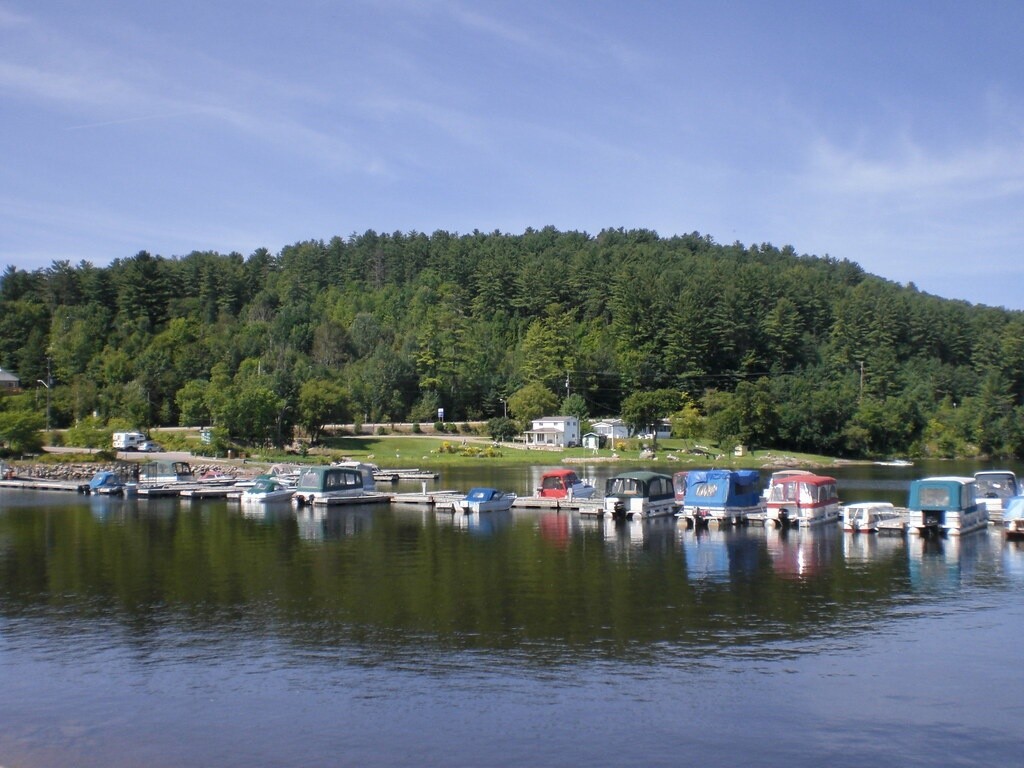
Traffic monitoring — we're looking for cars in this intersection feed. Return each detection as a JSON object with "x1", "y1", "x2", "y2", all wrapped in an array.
[{"x1": 138, "y1": 441, "x2": 164, "y2": 452}]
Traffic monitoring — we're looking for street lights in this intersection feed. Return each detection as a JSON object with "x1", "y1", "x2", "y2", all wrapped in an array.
[
  {"x1": 500, "y1": 398, "x2": 507, "y2": 418},
  {"x1": 37, "y1": 380, "x2": 49, "y2": 431}
]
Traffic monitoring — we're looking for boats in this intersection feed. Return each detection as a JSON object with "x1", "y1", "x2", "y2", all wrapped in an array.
[
  {"x1": 241, "y1": 499, "x2": 292, "y2": 525},
  {"x1": 603, "y1": 469, "x2": 1024, "y2": 535},
  {"x1": 292, "y1": 502, "x2": 392, "y2": 543},
  {"x1": 766, "y1": 521, "x2": 843, "y2": 587},
  {"x1": 874, "y1": 460, "x2": 914, "y2": 467},
  {"x1": 1000, "y1": 536, "x2": 1024, "y2": 586},
  {"x1": 603, "y1": 513, "x2": 675, "y2": 568},
  {"x1": 453, "y1": 487, "x2": 517, "y2": 513},
  {"x1": 534, "y1": 470, "x2": 596, "y2": 498},
  {"x1": 87, "y1": 460, "x2": 394, "y2": 507},
  {"x1": 909, "y1": 527, "x2": 998, "y2": 597},
  {"x1": 681, "y1": 524, "x2": 760, "y2": 606},
  {"x1": 453, "y1": 510, "x2": 517, "y2": 539},
  {"x1": 844, "y1": 529, "x2": 904, "y2": 574}
]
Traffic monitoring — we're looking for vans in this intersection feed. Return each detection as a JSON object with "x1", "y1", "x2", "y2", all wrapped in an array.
[{"x1": 112, "y1": 433, "x2": 146, "y2": 452}]
